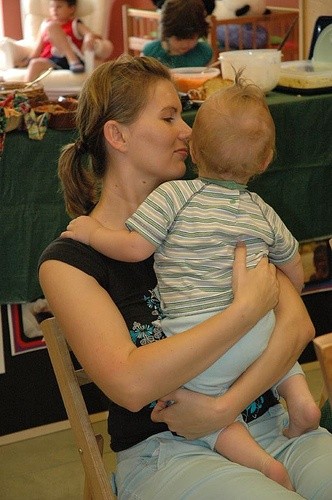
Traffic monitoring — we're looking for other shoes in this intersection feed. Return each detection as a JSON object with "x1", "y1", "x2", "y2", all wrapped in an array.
[{"x1": 70, "y1": 61, "x2": 84, "y2": 72}]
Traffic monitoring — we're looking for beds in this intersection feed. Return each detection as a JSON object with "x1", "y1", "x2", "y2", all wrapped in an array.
[{"x1": 122, "y1": 4, "x2": 300, "y2": 67}]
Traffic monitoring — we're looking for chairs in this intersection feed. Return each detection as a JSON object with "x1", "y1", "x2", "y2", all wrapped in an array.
[
  {"x1": 307, "y1": 15, "x2": 332, "y2": 63},
  {"x1": 39, "y1": 314, "x2": 115, "y2": 500},
  {"x1": 312, "y1": 329, "x2": 332, "y2": 411}
]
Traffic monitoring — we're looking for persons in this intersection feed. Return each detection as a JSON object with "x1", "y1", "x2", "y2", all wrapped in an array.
[
  {"x1": 139, "y1": 0, "x2": 213, "y2": 69},
  {"x1": 17, "y1": 0, "x2": 103, "y2": 83},
  {"x1": 38, "y1": 54, "x2": 332, "y2": 500},
  {"x1": 60, "y1": 63, "x2": 321, "y2": 492}
]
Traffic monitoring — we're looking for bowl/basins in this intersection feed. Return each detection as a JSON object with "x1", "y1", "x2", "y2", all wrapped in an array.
[
  {"x1": 0, "y1": 83, "x2": 44, "y2": 99},
  {"x1": 171, "y1": 67, "x2": 220, "y2": 93},
  {"x1": 279, "y1": 59, "x2": 332, "y2": 89},
  {"x1": 219, "y1": 48, "x2": 281, "y2": 93}
]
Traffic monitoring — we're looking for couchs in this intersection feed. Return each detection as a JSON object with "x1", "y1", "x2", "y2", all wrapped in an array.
[{"x1": 0, "y1": 0, "x2": 116, "y2": 94}]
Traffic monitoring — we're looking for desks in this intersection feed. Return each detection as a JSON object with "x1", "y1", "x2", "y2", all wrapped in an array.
[{"x1": 0, "y1": 90, "x2": 332, "y2": 437}]
